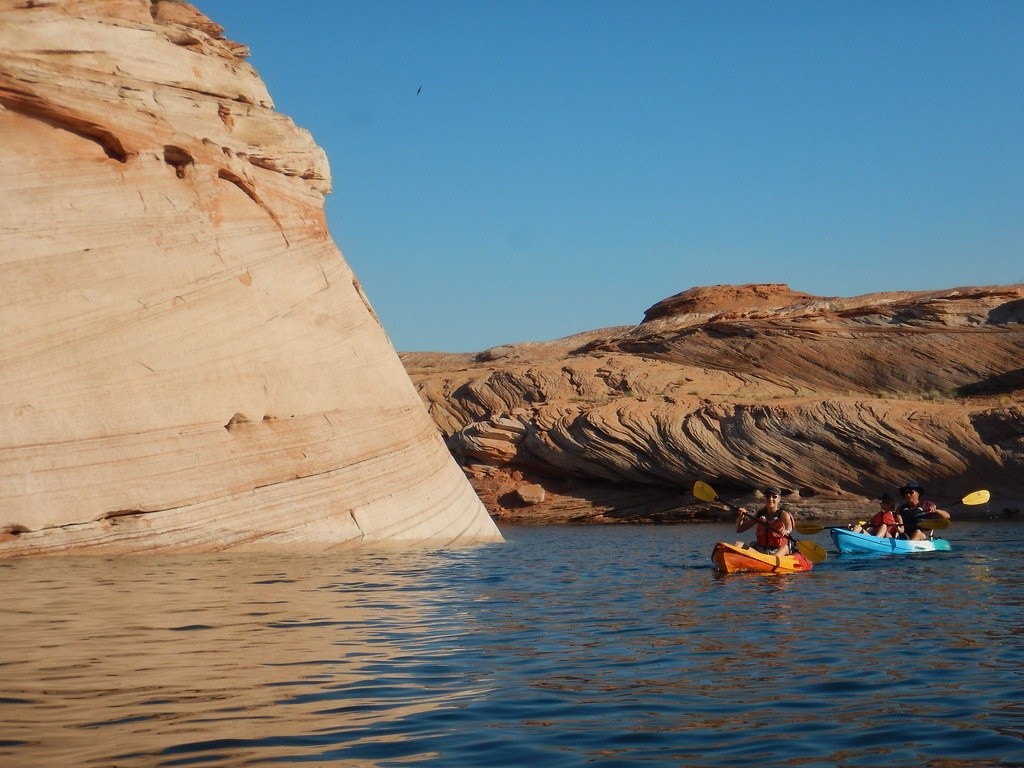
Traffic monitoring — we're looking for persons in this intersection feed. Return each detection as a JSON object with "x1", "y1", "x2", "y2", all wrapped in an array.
[
  {"x1": 735, "y1": 488, "x2": 795, "y2": 556},
  {"x1": 847, "y1": 481, "x2": 950, "y2": 542}
]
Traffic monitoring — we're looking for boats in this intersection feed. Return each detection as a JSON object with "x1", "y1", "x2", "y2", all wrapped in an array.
[
  {"x1": 830, "y1": 526, "x2": 953, "y2": 554},
  {"x1": 711, "y1": 541, "x2": 814, "y2": 576}
]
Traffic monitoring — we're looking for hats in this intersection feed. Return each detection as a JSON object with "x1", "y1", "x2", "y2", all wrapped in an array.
[
  {"x1": 877, "y1": 493, "x2": 897, "y2": 506},
  {"x1": 763, "y1": 487, "x2": 781, "y2": 496},
  {"x1": 899, "y1": 482, "x2": 924, "y2": 496}
]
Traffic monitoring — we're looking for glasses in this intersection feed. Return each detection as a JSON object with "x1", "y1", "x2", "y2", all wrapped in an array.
[
  {"x1": 903, "y1": 491, "x2": 916, "y2": 495},
  {"x1": 766, "y1": 495, "x2": 779, "y2": 499}
]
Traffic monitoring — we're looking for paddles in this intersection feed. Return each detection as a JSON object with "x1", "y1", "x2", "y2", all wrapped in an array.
[
  {"x1": 797, "y1": 515, "x2": 951, "y2": 535},
  {"x1": 693, "y1": 481, "x2": 828, "y2": 562},
  {"x1": 926, "y1": 490, "x2": 991, "y2": 509}
]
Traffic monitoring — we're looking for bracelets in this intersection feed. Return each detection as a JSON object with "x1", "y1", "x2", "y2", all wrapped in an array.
[
  {"x1": 785, "y1": 528, "x2": 790, "y2": 532},
  {"x1": 736, "y1": 521, "x2": 744, "y2": 526}
]
